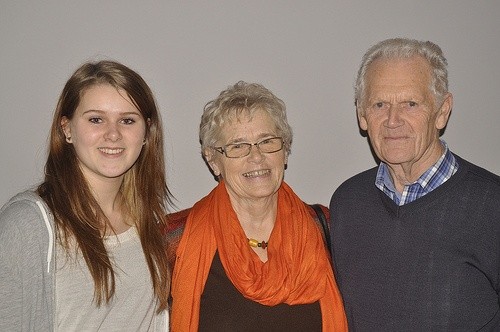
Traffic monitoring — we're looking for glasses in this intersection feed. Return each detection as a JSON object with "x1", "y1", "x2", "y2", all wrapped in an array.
[{"x1": 211, "y1": 137, "x2": 285, "y2": 159}]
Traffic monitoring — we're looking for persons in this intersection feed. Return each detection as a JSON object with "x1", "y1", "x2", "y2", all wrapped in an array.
[
  {"x1": 154, "y1": 80, "x2": 349, "y2": 332},
  {"x1": 329, "y1": 37, "x2": 500, "y2": 332},
  {"x1": 0, "y1": 59, "x2": 173, "y2": 332}
]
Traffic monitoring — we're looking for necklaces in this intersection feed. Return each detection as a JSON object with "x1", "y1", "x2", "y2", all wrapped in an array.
[{"x1": 246, "y1": 238, "x2": 269, "y2": 250}]
